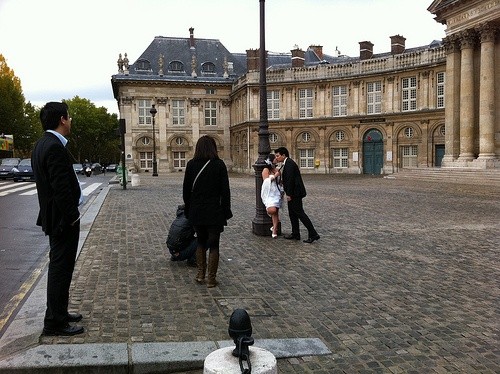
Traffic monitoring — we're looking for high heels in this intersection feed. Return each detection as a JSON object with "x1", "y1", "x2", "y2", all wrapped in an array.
[
  {"x1": 272, "y1": 232, "x2": 277, "y2": 238},
  {"x1": 270, "y1": 227, "x2": 273, "y2": 231}
]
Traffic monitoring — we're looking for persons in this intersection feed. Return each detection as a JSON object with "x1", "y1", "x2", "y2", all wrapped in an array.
[
  {"x1": 83, "y1": 159, "x2": 89, "y2": 171},
  {"x1": 166, "y1": 204, "x2": 197, "y2": 267},
  {"x1": 183, "y1": 136, "x2": 233, "y2": 287},
  {"x1": 274, "y1": 147, "x2": 320, "y2": 243},
  {"x1": 262, "y1": 154, "x2": 284, "y2": 238},
  {"x1": 101, "y1": 163, "x2": 106, "y2": 176},
  {"x1": 31, "y1": 102, "x2": 85, "y2": 335}
]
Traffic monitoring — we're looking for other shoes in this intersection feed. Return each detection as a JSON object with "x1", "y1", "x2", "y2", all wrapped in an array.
[
  {"x1": 170, "y1": 256, "x2": 182, "y2": 261},
  {"x1": 187, "y1": 261, "x2": 196, "y2": 267}
]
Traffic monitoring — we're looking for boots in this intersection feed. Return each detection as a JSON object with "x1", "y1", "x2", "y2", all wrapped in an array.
[
  {"x1": 195, "y1": 248, "x2": 207, "y2": 282},
  {"x1": 207, "y1": 250, "x2": 220, "y2": 288}
]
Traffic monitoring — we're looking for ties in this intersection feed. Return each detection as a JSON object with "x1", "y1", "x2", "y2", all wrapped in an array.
[{"x1": 276, "y1": 164, "x2": 284, "y2": 192}]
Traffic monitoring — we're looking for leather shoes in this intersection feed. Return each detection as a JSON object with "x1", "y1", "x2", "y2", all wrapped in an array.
[
  {"x1": 284, "y1": 235, "x2": 300, "y2": 240},
  {"x1": 303, "y1": 235, "x2": 320, "y2": 242},
  {"x1": 43, "y1": 326, "x2": 85, "y2": 336},
  {"x1": 67, "y1": 313, "x2": 83, "y2": 323}
]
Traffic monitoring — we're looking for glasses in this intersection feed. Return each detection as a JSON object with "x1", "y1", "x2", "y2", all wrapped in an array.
[{"x1": 64, "y1": 116, "x2": 73, "y2": 121}]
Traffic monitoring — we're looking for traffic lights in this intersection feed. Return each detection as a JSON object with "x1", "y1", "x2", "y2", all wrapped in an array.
[{"x1": 119, "y1": 119, "x2": 128, "y2": 191}]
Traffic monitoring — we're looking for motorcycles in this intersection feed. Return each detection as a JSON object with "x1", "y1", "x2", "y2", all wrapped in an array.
[{"x1": 84, "y1": 163, "x2": 92, "y2": 177}]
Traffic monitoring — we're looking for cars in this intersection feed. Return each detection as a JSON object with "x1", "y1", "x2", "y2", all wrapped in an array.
[
  {"x1": 106, "y1": 164, "x2": 118, "y2": 172},
  {"x1": 0, "y1": 158, "x2": 22, "y2": 179},
  {"x1": 73, "y1": 164, "x2": 83, "y2": 175},
  {"x1": 13, "y1": 158, "x2": 37, "y2": 183}
]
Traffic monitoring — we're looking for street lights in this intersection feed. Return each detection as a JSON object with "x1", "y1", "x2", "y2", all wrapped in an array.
[{"x1": 149, "y1": 104, "x2": 159, "y2": 176}]
